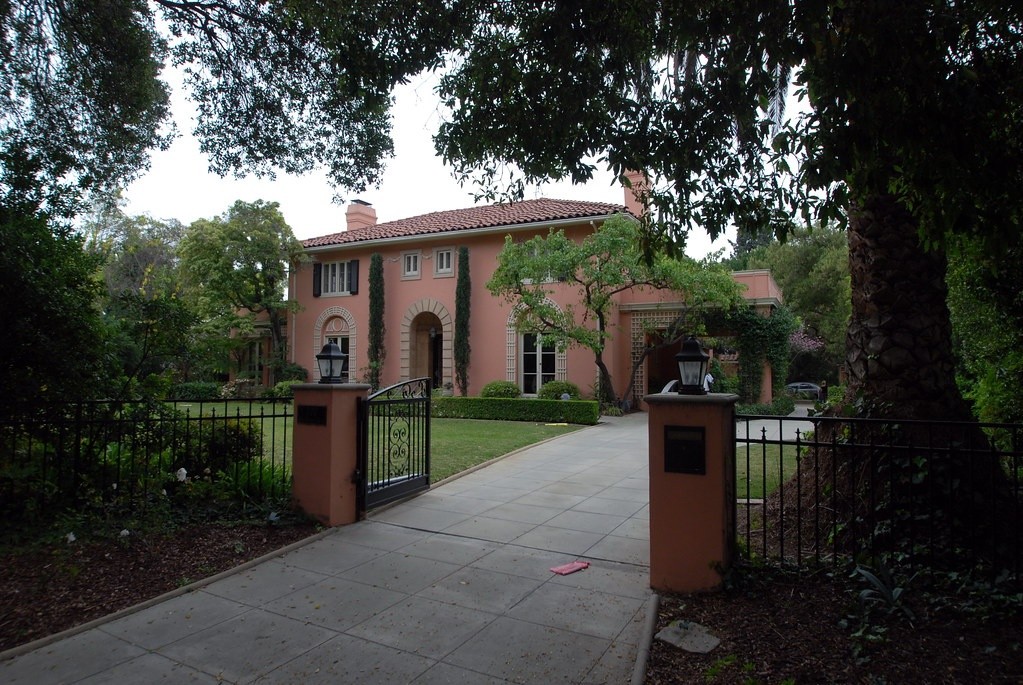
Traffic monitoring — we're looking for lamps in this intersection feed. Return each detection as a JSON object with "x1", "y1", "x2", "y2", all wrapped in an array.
[
  {"x1": 316, "y1": 339, "x2": 349, "y2": 384},
  {"x1": 429, "y1": 320, "x2": 438, "y2": 339},
  {"x1": 674, "y1": 335, "x2": 713, "y2": 394}
]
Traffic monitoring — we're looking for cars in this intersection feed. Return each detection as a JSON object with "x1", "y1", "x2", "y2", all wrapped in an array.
[{"x1": 785, "y1": 382, "x2": 823, "y2": 400}]
